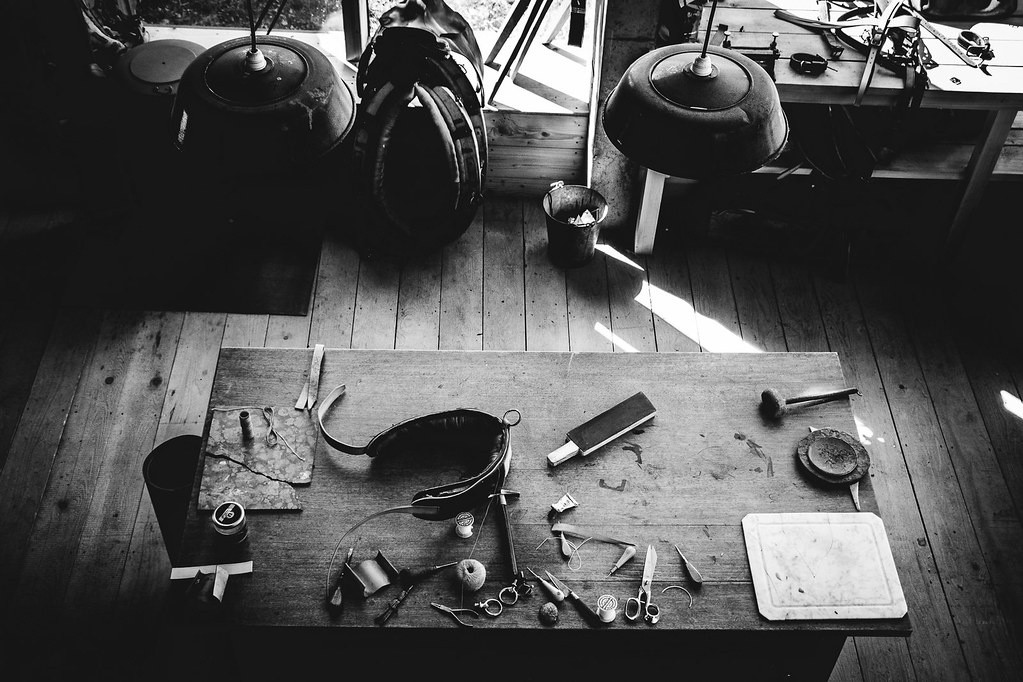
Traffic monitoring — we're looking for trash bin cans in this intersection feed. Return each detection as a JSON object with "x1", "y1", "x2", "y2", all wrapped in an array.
[
  {"x1": 141, "y1": 435, "x2": 205, "y2": 570},
  {"x1": 543, "y1": 185, "x2": 609, "y2": 266}
]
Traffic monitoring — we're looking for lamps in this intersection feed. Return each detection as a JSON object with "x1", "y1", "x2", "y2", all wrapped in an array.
[
  {"x1": 600, "y1": 0, "x2": 791, "y2": 182},
  {"x1": 168, "y1": 0, "x2": 359, "y2": 177}
]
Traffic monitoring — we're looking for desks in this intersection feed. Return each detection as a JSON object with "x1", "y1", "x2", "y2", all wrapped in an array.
[
  {"x1": 676, "y1": 0, "x2": 1023, "y2": 245},
  {"x1": 159, "y1": 346, "x2": 916, "y2": 682}
]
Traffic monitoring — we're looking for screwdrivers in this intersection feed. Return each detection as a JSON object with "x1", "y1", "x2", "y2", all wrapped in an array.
[
  {"x1": 527, "y1": 566, "x2": 564, "y2": 602},
  {"x1": 675, "y1": 545, "x2": 703, "y2": 583},
  {"x1": 606, "y1": 546, "x2": 637, "y2": 580}
]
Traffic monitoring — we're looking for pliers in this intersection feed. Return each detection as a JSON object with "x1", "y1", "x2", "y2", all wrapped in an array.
[{"x1": 430, "y1": 603, "x2": 479, "y2": 627}]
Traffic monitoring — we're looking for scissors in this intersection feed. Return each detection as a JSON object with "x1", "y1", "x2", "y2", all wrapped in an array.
[{"x1": 625, "y1": 545, "x2": 660, "y2": 625}]
